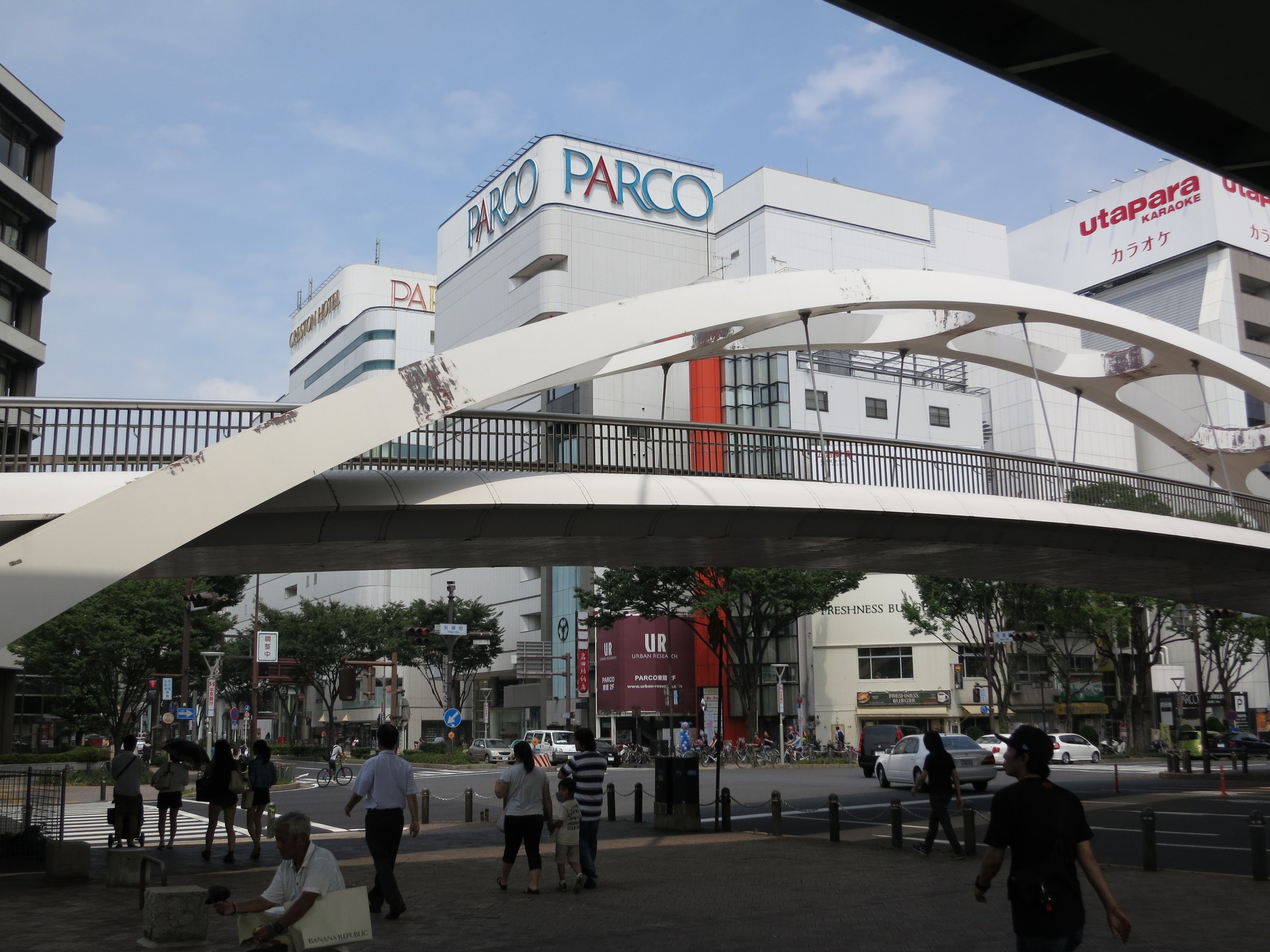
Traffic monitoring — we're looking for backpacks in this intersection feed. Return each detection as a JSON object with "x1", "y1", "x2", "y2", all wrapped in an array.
[{"x1": 323, "y1": 745, "x2": 339, "y2": 760}]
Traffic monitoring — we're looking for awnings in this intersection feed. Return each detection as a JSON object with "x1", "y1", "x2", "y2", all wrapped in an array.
[
  {"x1": 1054, "y1": 702, "x2": 1109, "y2": 715},
  {"x1": 961, "y1": 705, "x2": 1016, "y2": 724}
]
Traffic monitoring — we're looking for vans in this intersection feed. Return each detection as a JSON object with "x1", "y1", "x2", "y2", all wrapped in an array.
[
  {"x1": 524, "y1": 730, "x2": 580, "y2": 766},
  {"x1": 858, "y1": 724, "x2": 924, "y2": 778}
]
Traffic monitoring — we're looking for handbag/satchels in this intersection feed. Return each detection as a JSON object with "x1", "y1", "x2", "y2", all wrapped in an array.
[
  {"x1": 283, "y1": 885, "x2": 373, "y2": 952},
  {"x1": 155, "y1": 764, "x2": 174, "y2": 790},
  {"x1": 241, "y1": 779, "x2": 254, "y2": 809},
  {"x1": 195, "y1": 770, "x2": 209, "y2": 802},
  {"x1": 496, "y1": 811, "x2": 505, "y2": 833},
  {"x1": 352, "y1": 742, "x2": 356, "y2": 746},
  {"x1": 229, "y1": 763, "x2": 245, "y2": 794}
]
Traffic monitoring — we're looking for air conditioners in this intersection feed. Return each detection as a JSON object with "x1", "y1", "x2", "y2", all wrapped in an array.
[{"x1": 1011, "y1": 683, "x2": 1022, "y2": 695}]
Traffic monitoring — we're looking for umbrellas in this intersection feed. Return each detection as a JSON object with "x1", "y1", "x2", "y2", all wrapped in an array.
[
  {"x1": 160, "y1": 738, "x2": 211, "y2": 766},
  {"x1": 434, "y1": 737, "x2": 445, "y2": 744}
]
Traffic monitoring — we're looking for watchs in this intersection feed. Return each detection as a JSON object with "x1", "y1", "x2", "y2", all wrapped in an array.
[{"x1": 272, "y1": 920, "x2": 283, "y2": 935}]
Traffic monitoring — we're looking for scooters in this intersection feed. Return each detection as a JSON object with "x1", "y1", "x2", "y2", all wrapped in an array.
[{"x1": 1100, "y1": 740, "x2": 1117, "y2": 755}]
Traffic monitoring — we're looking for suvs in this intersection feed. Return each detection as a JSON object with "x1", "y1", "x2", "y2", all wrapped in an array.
[{"x1": 136, "y1": 737, "x2": 146, "y2": 750}]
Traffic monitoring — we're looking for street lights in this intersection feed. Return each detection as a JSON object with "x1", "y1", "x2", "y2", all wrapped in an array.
[
  {"x1": 771, "y1": 664, "x2": 787, "y2": 764},
  {"x1": 480, "y1": 688, "x2": 492, "y2": 738},
  {"x1": 200, "y1": 651, "x2": 225, "y2": 761},
  {"x1": 1171, "y1": 678, "x2": 1186, "y2": 726}
]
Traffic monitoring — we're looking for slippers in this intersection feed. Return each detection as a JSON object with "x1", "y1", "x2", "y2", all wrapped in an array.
[
  {"x1": 523, "y1": 888, "x2": 539, "y2": 895},
  {"x1": 497, "y1": 876, "x2": 507, "y2": 890}
]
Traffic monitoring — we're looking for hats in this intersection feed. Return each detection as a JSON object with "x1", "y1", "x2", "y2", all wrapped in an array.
[{"x1": 995, "y1": 724, "x2": 1053, "y2": 762}]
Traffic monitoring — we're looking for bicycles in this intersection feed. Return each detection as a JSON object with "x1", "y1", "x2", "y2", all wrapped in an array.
[
  {"x1": 611, "y1": 742, "x2": 651, "y2": 763},
  {"x1": 728, "y1": 739, "x2": 859, "y2": 769},
  {"x1": 317, "y1": 757, "x2": 353, "y2": 788},
  {"x1": 672, "y1": 742, "x2": 727, "y2": 768}
]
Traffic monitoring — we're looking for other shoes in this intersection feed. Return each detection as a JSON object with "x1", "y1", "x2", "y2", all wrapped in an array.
[
  {"x1": 331, "y1": 780, "x2": 336, "y2": 784},
  {"x1": 224, "y1": 854, "x2": 234, "y2": 862},
  {"x1": 556, "y1": 882, "x2": 566, "y2": 892},
  {"x1": 950, "y1": 853, "x2": 965, "y2": 859},
  {"x1": 127, "y1": 843, "x2": 138, "y2": 848},
  {"x1": 167, "y1": 842, "x2": 173, "y2": 848},
  {"x1": 202, "y1": 850, "x2": 210, "y2": 860},
  {"x1": 385, "y1": 903, "x2": 407, "y2": 919},
  {"x1": 367, "y1": 900, "x2": 381, "y2": 913},
  {"x1": 157, "y1": 842, "x2": 165, "y2": 849},
  {"x1": 250, "y1": 846, "x2": 262, "y2": 859},
  {"x1": 574, "y1": 875, "x2": 588, "y2": 893},
  {"x1": 584, "y1": 880, "x2": 597, "y2": 889},
  {"x1": 115, "y1": 844, "x2": 123, "y2": 848},
  {"x1": 912, "y1": 843, "x2": 930, "y2": 858}
]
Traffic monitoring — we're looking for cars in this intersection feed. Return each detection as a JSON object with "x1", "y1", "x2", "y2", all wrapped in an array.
[
  {"x1": 1047, "y1": 734, "x2": 1100, "y2": 765},
  {"x1": 508, "y1": 740, "x2": 541, "y2": 757},
  {"x1": 1174, "y1": 731, "x2": 1270, "y2": 761},
  {"x1": 975, "y1": 734, "x2": 1011, "y2": 765},
  {"x1": 468, "y1": 739, "x2": 516, "y2": 765},
  {"x1": 875, "y1": 733, "x2": 997, "y2": 793},
  {"x1": 595, "y1": 740, "x2": 621, "y2": 768}
]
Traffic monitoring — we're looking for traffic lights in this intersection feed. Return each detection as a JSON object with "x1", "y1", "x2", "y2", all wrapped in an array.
[
  {"x1": 147, "y1": 678, "x2": 160, "y2": 700},
  {"x1": 1008, "y1": 632, "x2": 1034, "y2": 642},
  {"x1": 973, "y1": 688, "x2": 980, "y2": 703},
  {"x1": 377, "y1": 714, "x2": 382, "y2": 723},
  {"x1": 414, "y1": 638, "x2": 430, "y2": 646},
  {"x1": 306, "y1": 718, "x2": 310, "y2": 725},
  {"x1": 405, "y1": 627, "x2": 431, "y2": 637},
  {"x1": 183, "y1": 593, "x2": 219, "y2": 612}
]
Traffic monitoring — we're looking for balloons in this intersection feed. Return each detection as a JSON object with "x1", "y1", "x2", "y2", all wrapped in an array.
[
  {"x1": 699, "y1": 729, "x2": 705, "y2": 742},
  {"x1": 679, "y1": 721, "x2": 692, "y2": 752}
]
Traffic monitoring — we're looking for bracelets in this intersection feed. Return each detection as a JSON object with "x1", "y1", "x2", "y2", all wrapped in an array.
[
  {"x1": 231, "y1": 902, "x2": 237, "y2": 917},
  {"x1": 348, "y1": 801, "x2": 354, "y2": 808}
]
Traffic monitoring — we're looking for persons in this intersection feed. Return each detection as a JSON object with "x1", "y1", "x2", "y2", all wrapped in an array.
[
  {"x1": 546, "y1": 777, "x2": 588, "y2": 894},
  {"x1": 215, "y1": 812, "x2": 359, "y2": 952},
  {"x1": 151, "y1": 754, "x2": 189, "y2": 850},
  {"x1": 328, "y1": 723, "x2": 420, "y2": 920},
  {"x1": 245, "y1": 732, "x2": 278, "y2": 859},
  {"x1": 201, "y1": 731, "x2": 249, "y2": 862},
  {"x1": 417, "y1": 737, "x2": 424, "y2": 750},
  {"x1": 708, "y1": 733, "x2": 722, "y2": 758},
  {"x1": 974, "y1": 724, "x2": 1133, "y2": 952},
  {"x1": 751, "y1": 731, "x2": 802, "y2": 752},
  {"x1": 495, "y1": 741, "x2": 554, "y2": 894},
  {"x1": 467, "y1": 738, "x2": 473, "y2": 749},
  {"x1": 558, "y1": 728, "x2": 607, "y2": 889},
  {"x1": 912, "y1": 730, "x2": 965, "y2": 860},
  {"x1": 835, "y1": 726, "x2": 844, "y2": 748},
  {"x1": 111, "y1": 734, "x2": 152, "y2": 849}
]
